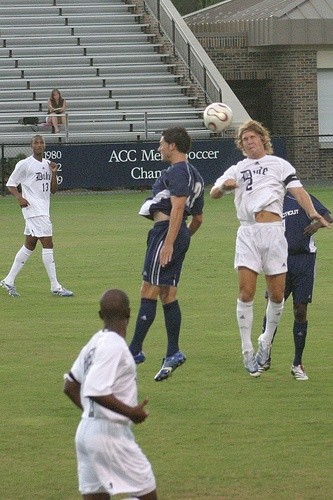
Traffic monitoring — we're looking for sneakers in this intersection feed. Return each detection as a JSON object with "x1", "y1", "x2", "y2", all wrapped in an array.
[
  {"x1": 250, "y1": 355, "x2": 272, "y2": 377},
  {"x1": 255, "y1": 334, "x2": 272, "y2": 366},
  {"x1": 0, "y1": 280, "x2": 20, "y2": 298},
  {"x1": 50, "y1": 285, "x2": 74, "y2": 297},
  {"x1": 154, "y1": 351, "x2": 187, "y2": 383},
  {"x1": 133, "y1": 351, "x2": 147, "y2": 364},
  {"x1": 290, "y1": 363, "x2": 308, "y2": 381},
  {"x1": 242, "y1": 349, "x2": 258, "y2": 374}
]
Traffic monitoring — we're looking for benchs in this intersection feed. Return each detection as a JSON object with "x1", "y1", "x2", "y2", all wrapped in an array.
[{"x1": 0, "y1": 1, "x2": 225, "y2": 141}]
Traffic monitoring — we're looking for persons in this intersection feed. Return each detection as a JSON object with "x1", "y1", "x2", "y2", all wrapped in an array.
[
  {"x1": 130, "y1": 126, "x2": 204, "y2": 381},
  {"x1": 0, "y1": 135, "x2": 74, "y2": 297},
  {"x1": 261, "y1": 190, "x2": 333, "y2": 381},
  {"x1": 210, "y1": 121, "x2": 332, "y2": 377},
  {"x1": 46, "y1": 89, "x2": 69, "y2": 134},
  {"x1": 63, "y1": 289, "x2": 157, "y2": 500}
]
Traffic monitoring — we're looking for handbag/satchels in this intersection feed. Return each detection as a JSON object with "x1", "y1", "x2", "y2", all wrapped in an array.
[{"x1": 23, "y1": 117, "x2": 41, "y2": 126}]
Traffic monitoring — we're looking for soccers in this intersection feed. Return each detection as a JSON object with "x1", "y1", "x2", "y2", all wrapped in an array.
[{"x1": 203, "y1": 102, "x2": 234, "y2": 134}]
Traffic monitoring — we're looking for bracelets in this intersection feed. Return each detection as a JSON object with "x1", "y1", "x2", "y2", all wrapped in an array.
[{"x1": 218, "y1": 184, "x2": 227, "y2": 194}]
